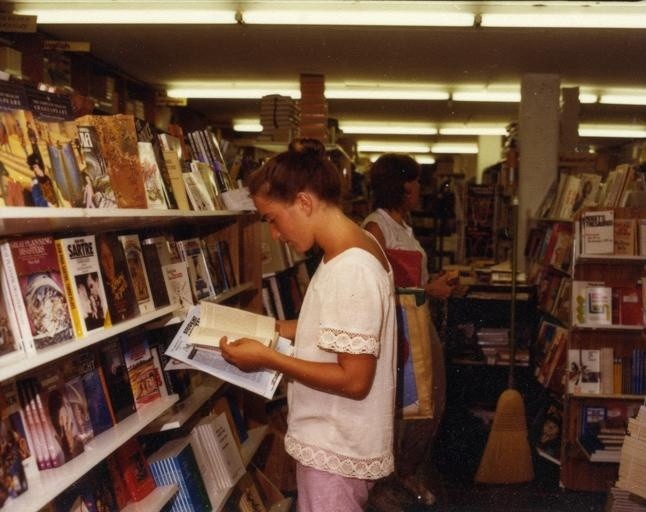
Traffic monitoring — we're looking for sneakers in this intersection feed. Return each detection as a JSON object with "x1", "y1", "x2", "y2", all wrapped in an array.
[{"x1": 368, "y1": 475, "x2": 435, "y2": 512}]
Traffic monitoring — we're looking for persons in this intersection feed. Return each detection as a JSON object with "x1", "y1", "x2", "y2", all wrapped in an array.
[{"x1": 222, "y1": 137, "x2": 454, "y2": 512}]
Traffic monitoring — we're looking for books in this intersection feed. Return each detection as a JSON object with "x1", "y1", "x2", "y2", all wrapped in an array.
[
  {"x1": 411, "y1": 146, "x2": 644, "y2": 510},
  {"x1": 2, "y1": 44, "x2": 364, "y2": 511}
]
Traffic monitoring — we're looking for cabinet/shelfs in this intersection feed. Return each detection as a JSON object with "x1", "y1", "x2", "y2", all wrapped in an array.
[{"x1": 1, "y1": 202, "x2": 271, "y2": 512}]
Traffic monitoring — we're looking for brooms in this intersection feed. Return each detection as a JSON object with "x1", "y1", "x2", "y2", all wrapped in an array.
[{"x1": 474, "y1": 196, "x2": 538, "y2": 483}]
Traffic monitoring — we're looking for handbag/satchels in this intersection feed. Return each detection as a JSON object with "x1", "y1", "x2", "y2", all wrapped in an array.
[{"x1": 384, "y1": 248, "x2": 423, "y2": 287}]
